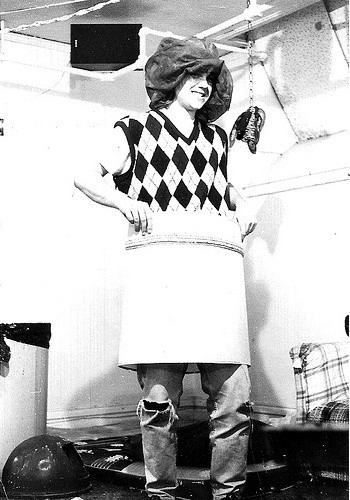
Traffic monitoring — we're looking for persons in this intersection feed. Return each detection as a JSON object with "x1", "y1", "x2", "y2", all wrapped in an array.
[{"x1": 74, "y1": 36, "x2": 257, "y2": 500}]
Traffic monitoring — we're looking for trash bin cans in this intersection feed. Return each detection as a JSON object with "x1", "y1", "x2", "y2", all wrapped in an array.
[{"x1": 0, "y1": 323, "x2": 51, "y2": 496}]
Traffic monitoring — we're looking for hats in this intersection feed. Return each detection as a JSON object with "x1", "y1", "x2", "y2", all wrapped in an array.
[{"x1": 144, "y1": 35, "x2": 234, "y2": 124}]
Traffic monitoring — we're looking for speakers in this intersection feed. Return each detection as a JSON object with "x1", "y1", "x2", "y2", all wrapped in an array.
[{"x1": 70, "y1": 23, "x2": 144, "y2": 72}]
